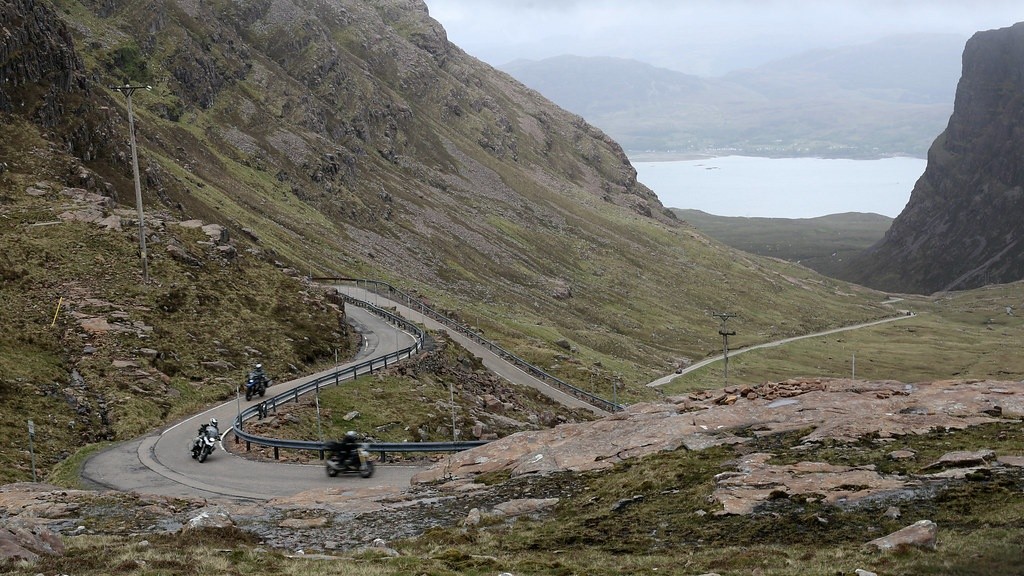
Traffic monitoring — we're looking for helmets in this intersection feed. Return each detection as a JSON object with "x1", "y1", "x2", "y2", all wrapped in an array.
[
  {"x1": 256, "y1": 364, "x2": 262, "y2": 371},
  {"x1": 347, "y1": 431, "x2": 358, "y2": 438},
  {"x1": 210, "y1": 418, "x2": 218, "y2": 426}
]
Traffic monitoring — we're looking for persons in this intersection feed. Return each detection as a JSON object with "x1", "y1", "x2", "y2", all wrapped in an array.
[
  {"x1": 246, "y1": 363, "x2": 266, "y2": 390},
  {"x1": 196, "y1": 417, "x2": 221, "y2": 454},
  {"x1": 339, "y1": 431, "x2": 360, "y2": 469}
]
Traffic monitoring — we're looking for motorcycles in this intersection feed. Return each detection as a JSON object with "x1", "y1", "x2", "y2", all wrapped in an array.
[
  {"x1": 190, "y1": 425, "x2": 224, "y2": 463},
  {"x1": 323, "y1": 444, "x2": 375, "y2": 478},
  {"x1": 245, "y1": 371, "x2": 273, "y2": 401}
]
